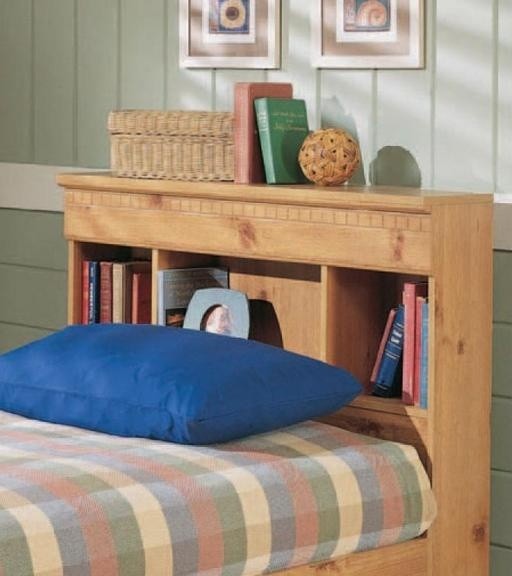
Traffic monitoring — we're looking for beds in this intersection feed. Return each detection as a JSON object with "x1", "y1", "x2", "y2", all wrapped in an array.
[{"x1": 1, "y1": 171, "x2": 493, "y2": 575}]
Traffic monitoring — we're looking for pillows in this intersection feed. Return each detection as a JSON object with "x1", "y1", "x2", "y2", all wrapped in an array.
[{"x1": 1, "y1": 324, "x2": 367, "y2": 446}]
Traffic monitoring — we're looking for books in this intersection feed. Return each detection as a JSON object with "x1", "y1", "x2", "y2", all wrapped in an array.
[
  {"x1": 81, "y1": 257, "x2": 151, "y2": 324},
  {"x1": 367, "y1": 276, "x2": 426, "y2": 408},
  {"x1": 253, "y1": 96, "x2": 316, "y2": 185},
  {"x1": 158, "y1": 266, "x2": 229, "y2": 327},
  {"x1": 232, "y1": 80, "x2": 292, "y2": 184}
]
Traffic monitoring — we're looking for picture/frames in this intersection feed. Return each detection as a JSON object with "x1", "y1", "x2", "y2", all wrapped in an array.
[
  {"x1": 310, "y1": 0, "x2": 427, "y2": 70},
  {"x1": 178, "y1": 0, "x2": 282, "y2": 71},
  {"x1": 181, "y1": 287, "x2": 251, "y2": 342}
]
